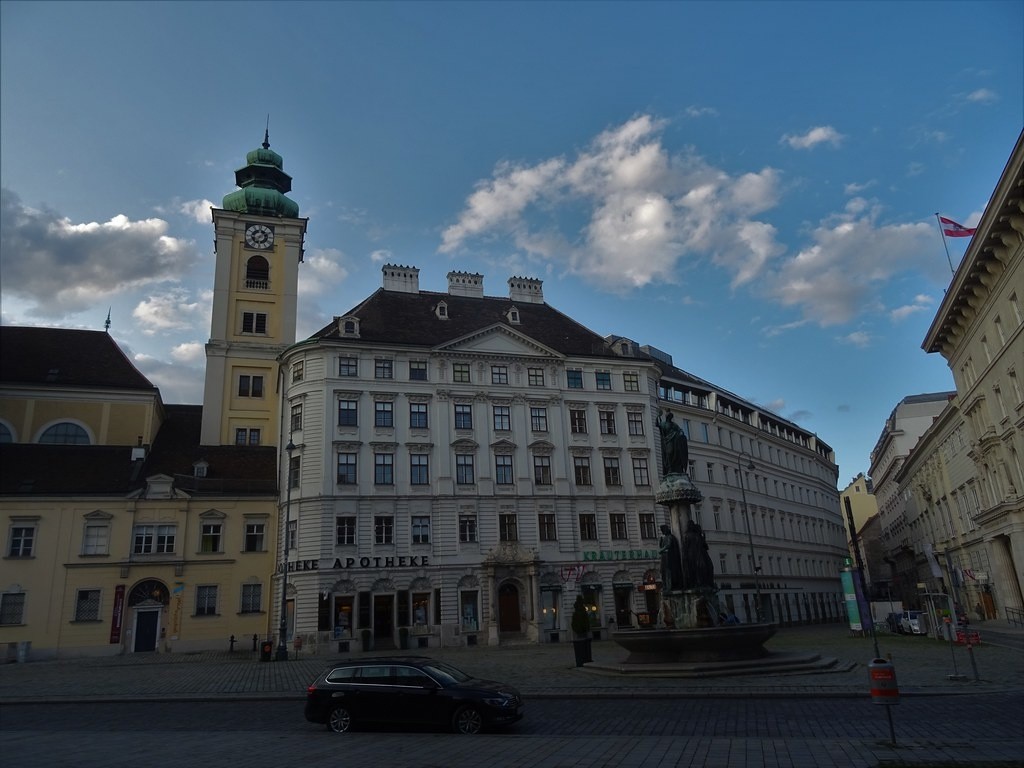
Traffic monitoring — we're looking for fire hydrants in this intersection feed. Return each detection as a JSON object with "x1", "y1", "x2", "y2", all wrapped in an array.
[
  {"x1": 228, "y1": 634, "x2": 237, "y2": 653},
  {"x1": 974, "y1": 602, "x2": 985, "y2": 623},
  {"x1": 251, "y1": 633, "x2": 259, "y2": 652}
]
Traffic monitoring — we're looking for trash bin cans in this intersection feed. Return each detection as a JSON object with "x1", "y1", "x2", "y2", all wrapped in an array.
[{"x1": 261, "y1": 641, "x2": 274, "y2": 663}]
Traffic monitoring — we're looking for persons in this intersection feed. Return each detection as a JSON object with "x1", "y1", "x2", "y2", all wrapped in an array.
[
  {"x1": 683, "y1": 520, "x2": 719, "y2": 588},
  {"x1": 955, "y1": 602, "x2": 959, "y2": 614},
  {"x1": 656, "y1": 524, "x2": 683, "y2": 588},
  {"x1": 719, "y1": 608, "x2": 740, "y2": 623},
  {"x1": 975, "y1": 602, "x2": 983, "y2": 623},
  {"x1": 935, "y1": 608, "x2": 944, "y2": 625},
  {"x1": 655, "y1": 409, "x2": 688, "y2": 475}
]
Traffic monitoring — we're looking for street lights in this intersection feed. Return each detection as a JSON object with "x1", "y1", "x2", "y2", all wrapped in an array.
[{"x1": 738, "y1": 451, "x2": 766, "y2": 622}]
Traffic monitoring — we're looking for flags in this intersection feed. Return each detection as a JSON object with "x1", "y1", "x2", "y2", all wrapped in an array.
[
  {"x1": 966, "y1": 570, "x2": 976, "y2": 580},
  {"x1": 940, "y1": 216, "x2": 978, "y2": 237}
]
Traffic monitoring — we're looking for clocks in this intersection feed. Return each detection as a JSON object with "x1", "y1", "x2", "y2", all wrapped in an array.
[{"x1": 246, "y1": 225, "x2": 274, "y2": 249}]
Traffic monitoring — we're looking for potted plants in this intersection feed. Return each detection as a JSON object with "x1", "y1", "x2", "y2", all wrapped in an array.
[{"x1": 570, "y1": 594, "x2": 593, "y2": 667}]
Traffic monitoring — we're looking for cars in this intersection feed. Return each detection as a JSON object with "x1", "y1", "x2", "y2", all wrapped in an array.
[
  {"x1": 303, "y1": 654, "x2": 527, "y2": 736},
  {"x1": 900, "y1": 610, "x2": 926, "y2": 635}
]
